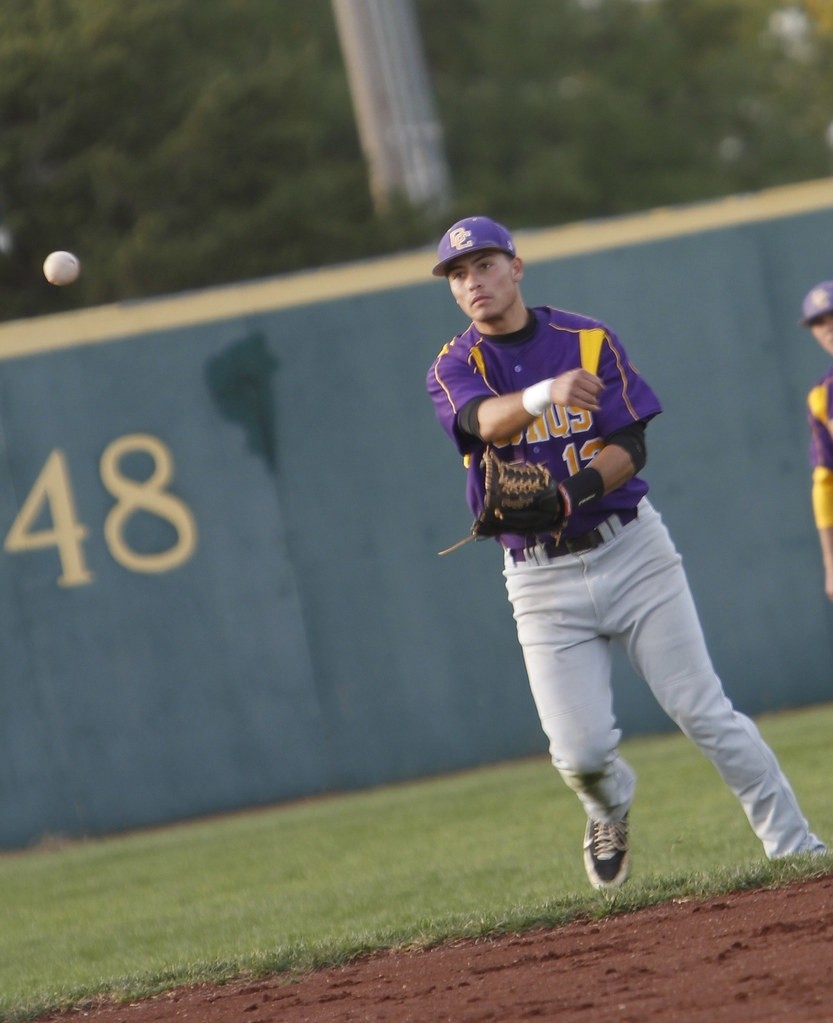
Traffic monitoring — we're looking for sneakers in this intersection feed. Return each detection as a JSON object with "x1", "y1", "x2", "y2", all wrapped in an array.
[{"x1": 582, "y1": 808, "x2": 631, "y2": 890}]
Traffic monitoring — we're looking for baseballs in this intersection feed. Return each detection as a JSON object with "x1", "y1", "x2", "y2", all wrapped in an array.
[{"x1": 43, "y1": 250, "x2": 80, "y2": 287}]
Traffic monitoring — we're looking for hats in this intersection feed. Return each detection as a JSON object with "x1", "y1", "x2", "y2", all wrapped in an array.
[
  {"x1": 795, "y1": 279, "x2": 833, "y2": 325},
  {"x1": 428, "y1": 216, "x2": 515, "y2": 278}
]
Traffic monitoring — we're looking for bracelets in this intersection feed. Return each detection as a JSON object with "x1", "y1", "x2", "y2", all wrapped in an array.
[{"x1": 522, "y1": 379, "x2": 554, "y2": 417}]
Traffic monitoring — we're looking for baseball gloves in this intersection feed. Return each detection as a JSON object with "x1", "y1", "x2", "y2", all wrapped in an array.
[{"x1": 471, "y1": 448, "x2": 561, "y2": 537}]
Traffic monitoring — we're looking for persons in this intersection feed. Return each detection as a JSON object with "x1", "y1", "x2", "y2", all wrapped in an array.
[
  {"x1": 798, "y1": 278, "x2": 833, "y2": 601},
  {"x1": 426, "y1": 216, "x2": 827, "y2": 891}
]
